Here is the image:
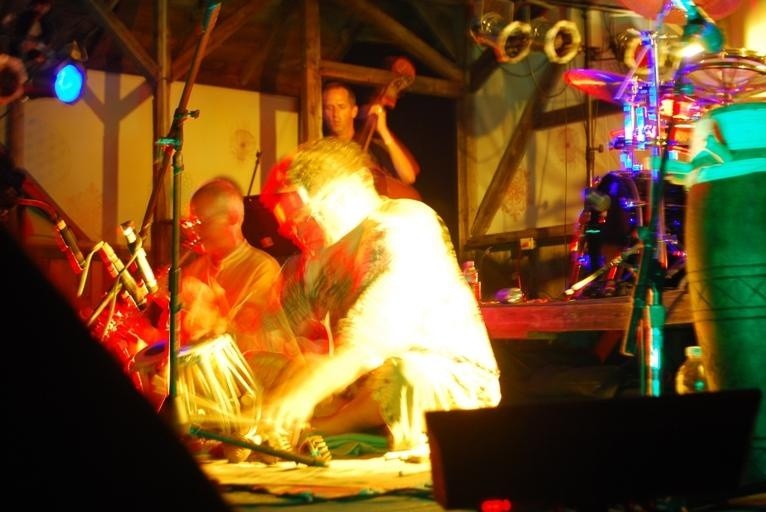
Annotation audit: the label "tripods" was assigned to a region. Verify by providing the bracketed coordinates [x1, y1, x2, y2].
[141, 0, 326, 470]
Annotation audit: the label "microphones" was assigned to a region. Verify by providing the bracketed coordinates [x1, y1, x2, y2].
[98, 241, 147, 312]
[52, 217, 86, 270]
[121, 226, 159, 295]
[674, 0, 726, 54]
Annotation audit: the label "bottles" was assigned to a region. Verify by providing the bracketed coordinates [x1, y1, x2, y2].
[676, 345, 714, 394]
[637, 288, 667, 397]
[464, 259, 478, 303]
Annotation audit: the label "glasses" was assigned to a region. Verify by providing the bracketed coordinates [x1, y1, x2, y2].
[183, 213, 227, 229]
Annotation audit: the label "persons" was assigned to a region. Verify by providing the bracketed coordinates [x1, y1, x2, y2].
[256, 140, 501, 457]
[322, 83, 415, 187]
[179, 179, 302, 361]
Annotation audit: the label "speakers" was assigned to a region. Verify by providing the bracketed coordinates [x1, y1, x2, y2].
[424, 386, 762, 512]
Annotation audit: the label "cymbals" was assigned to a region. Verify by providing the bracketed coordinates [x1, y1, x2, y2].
[565, 68, 646, 105]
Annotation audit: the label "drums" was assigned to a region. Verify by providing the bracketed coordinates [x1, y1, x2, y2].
[677, 47, 762, 101]
[134, 332, 260, 460]
[576, 173, 687, 297]
[688, 104, 766, 481]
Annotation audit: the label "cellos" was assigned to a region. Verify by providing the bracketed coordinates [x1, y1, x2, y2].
[359, 58, 422, 204]
